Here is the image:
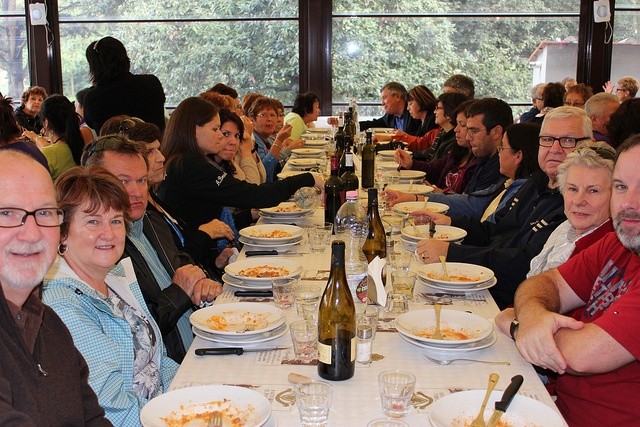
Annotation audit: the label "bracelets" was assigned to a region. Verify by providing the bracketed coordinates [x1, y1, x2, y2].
[414, 194, 418, 201]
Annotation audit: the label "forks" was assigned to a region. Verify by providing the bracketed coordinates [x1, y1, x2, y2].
[420, 293, 486, 301]
[397, 153, 402, 172]
[208, 414, 222, 427]
[423, 354, 510, 366]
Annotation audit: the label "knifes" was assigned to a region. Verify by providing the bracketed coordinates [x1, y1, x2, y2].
[429, 218, 437, 239]
[425, 292, 465, 297]
[486, 375, 524, 427]
[196, 346, 290, 355]
[245, 250, 295, 256]
[234, 290, 292, 297]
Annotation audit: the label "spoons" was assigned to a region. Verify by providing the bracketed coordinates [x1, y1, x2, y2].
[439, 256, 450, 281]
[432, 304, 444, 340]
[470, 373, 499, 427]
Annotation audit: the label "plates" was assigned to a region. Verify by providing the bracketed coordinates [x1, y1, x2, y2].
[416, 274, 497, 295]
[224, 257, 303, 285]
[427, 390, 566, 427]
[400, 225, 467, 241]
[287, 158, 322, 166]
[292, 153, 322, 158]
[393, 202, 450, 214]
[238, 236, 303, 251]
[377, 154, 413, 160]
[417, 262, 495, 288]
[139, 384, 272, 427]
[303, 144, 326, 147]
[259, 202, 311, 218]
[394, 309, 494, 347]
[307, 128, 331, 132]
[276, 171, 320, 180]
[398, 328, 497, 353]
[372, 132, 397, 141]
[191, 322, 290, 343]
[291, 149, 324, 154]
[377, 150, 413, 156]
[288, 165, 321, 169]
[387, 184, 434, 195]
[189, 302, 286, 336]
[384, 169, 426, 178]
[376, 141, 408, 145]
[258, 213, 309, 224]
[399, 235, 465, 244]
[239, 224, 305, 244]
[303, 140, 329, 144]
[222, 272, 301, 292]
[366, 127, 395, 133]
[378, 161, 401, 170]
[393, 211, 448, 218]
[392, 176, 426, 184]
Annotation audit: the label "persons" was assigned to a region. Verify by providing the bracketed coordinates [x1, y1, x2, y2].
[153, 97, 324, 250]
[21, 86, 99, 149]
[495, 135, 640, 427]
[99, 115, 238, 287]
[0, 94, 51, 178]
[563, 83, 593, 108]
[0, 148, 115, 427]
[282, 93, 321, 146]
[410, 92, 469, 164]
[80, 134, 222, 362]
[584, 92, 621, 149]
[560, 77, 577, 93]
[41, 166, 182, 427]
[200, 92, 267, 213]
[38, 94, 84, 183]
[528, 82, 565, 122]
[209, 83, 245, 117]
[525, 140, 619, 281]
[327, 83, 410, 131]
[83, 37, 165, 138]
[405, 85, 440, 137]
[416, 105, 593, 311]
[268, 100, 305, 163]
[380, 123, 541, 242]
[247, 97, 293, 184]
[13, 85, 49, 135]
[520, 83, 548, 123]
[460, 98, 515, 195]
[602, 77, 638, 104]
[391, 74, 475, 152]
[607, 98, 639, 149]
[206, 108, 261, 252]
[394, 99, 481, 195]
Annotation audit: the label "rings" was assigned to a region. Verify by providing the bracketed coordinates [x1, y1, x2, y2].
[421, 253, 426, 261]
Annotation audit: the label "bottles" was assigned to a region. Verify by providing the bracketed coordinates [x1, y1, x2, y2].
[324, 156, 344, 231]
[349, 107, 354, 114]
[340, 152, 359, 202]
[358, 187, 386, 287]
[293, 185, 324, 209]
[335, 112, 348, 151]
[385, 239, 395, 265]
[349, 112, 356, 137]
[334, 190, 370, 315]
[343, 114, 354, 145]
[339, 136, 355, 178]
[355, 326, 373, 362]
[362, 131, 374, 188]
[317, 240, 356, 381]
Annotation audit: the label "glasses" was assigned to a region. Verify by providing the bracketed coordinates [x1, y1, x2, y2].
[563, 100, 585, 107]
[538, 135, 590, 148]
[591, 145, 617, 161]
[496, 145, 512, 151]
[256, 112, 279, 118]
[616, 88, 627, 92]
[119, 116, 145, 136]
[435, 106, 444, 110]
[84, 136, 115, 165]
[0, 207, 66, 228]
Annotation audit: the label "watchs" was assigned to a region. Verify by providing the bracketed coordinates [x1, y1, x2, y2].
[510, 317, 520, 342]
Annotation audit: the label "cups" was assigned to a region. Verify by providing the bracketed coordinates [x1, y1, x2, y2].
[384, 291, 409, 315]
[355, 305, 379, 341]
[271, 277, 296, 310]
[366, 417, 410, 427]
[390, 270, 417, 300]
[289, 320, 320, 362]
[378, 370, 417, 418]
[294, 284, 321, 321]
[389, 251, 413, 274]
[295, 380, 333, 427]
[314, 222, 333, 245]
[307, 227, 326, 254]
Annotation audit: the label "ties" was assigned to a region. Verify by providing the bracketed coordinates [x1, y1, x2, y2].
[480, 178, 513, 222]
